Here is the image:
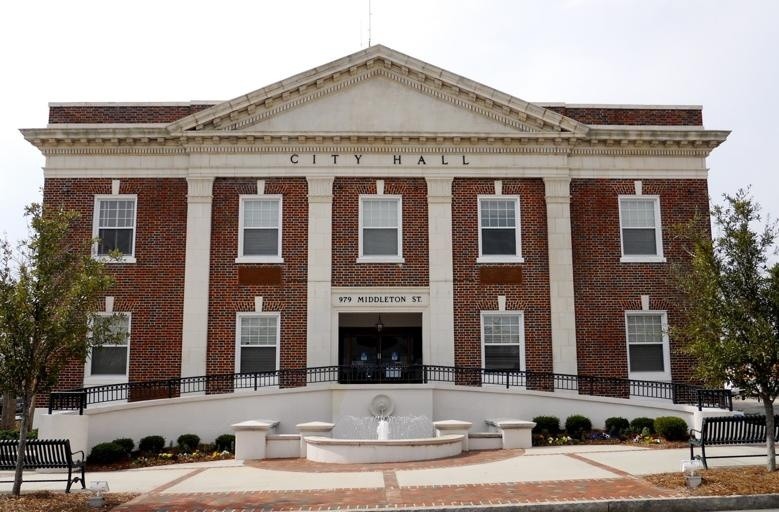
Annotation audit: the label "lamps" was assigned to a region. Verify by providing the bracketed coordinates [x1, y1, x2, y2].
[375, 313, 384, 332]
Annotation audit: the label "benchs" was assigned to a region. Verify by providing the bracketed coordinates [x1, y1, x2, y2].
[0, 439, 85, 493]
[689, 415, 778, 470]
